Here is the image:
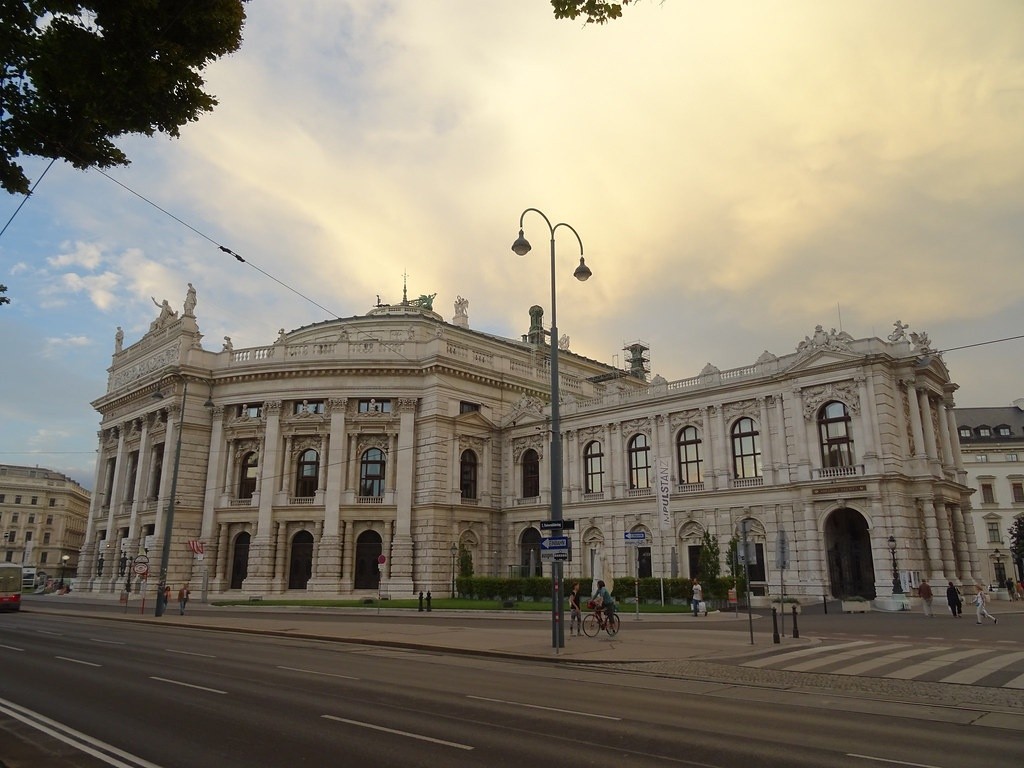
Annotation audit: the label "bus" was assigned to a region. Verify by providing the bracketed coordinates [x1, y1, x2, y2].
[0, 562, 23, 610]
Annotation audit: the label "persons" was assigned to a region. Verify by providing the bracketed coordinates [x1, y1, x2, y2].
[178, 585, 191, 616]
[590, 580, 616, 629]
[692, 578, 707, 617]
[970, 584, 998, 625]
[918, 579, 936, 618]
[151, 295, 175, 330]
[1005, 577, 1024, 602]
[51, 579, 70, 593]
[568, 582, 584, 637]
[946, 582, 962, 618]
[183, 282, 197, 316]
[114, 326, 124, 355]
[277, 328, 287, 345]
[454, 297, 469, 316]
[223, 337, 233, 352]
[161, 585, 170, 615]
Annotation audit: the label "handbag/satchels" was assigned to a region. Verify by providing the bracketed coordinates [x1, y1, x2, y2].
[691, 604, 693, 610]
[699, 602, 706, 612]
[955, 588, 963, 601]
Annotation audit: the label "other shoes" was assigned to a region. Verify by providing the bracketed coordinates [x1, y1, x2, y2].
[994, 619, 998, 624]
[976, 622, 982, 625]
[692, 614, 697, 616]
[705, 612, 707, 616]
[577, 633, 584, 636]
[570, 634, 577, 637]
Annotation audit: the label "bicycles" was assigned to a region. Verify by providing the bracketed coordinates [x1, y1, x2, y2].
[582, 602, 621, 638]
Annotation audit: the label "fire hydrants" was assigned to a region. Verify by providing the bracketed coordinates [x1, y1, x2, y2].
[426, 591, 433, 612]
[418, 591, 424, 612]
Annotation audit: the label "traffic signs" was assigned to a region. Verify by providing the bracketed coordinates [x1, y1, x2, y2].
[539, 537, 567, 549]
[624, 532, 646, 540]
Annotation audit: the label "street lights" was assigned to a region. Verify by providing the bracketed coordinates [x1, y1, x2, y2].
[152, 371, 216, 617]
[1001, 535, 1018, 583]
[887, 535, 904, 595]
[450, 542, 458, 599]
[56, 555, 70, 590]
[994, 548, 1007, 589]
[513, 209, 593, 645]
[124, 556, 133, 592]
